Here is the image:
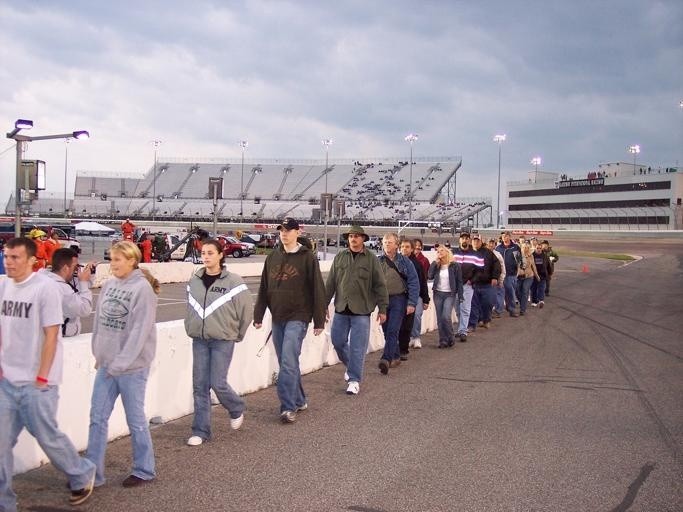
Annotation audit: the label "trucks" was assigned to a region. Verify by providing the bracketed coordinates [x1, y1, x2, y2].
[28, 228, 80, 255]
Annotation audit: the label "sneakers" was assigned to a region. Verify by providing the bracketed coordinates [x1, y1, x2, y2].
[468, 294, 551, 334]
[64, 463, 99, 506]
[437, 340, 455, 349]
[279, 402, 308, 425]
[185, 434, 207, 447]
[454, 333, 468, 343]
[343, 368, 361, 395]
[378, 352, 409, 376]
[229, 413, 244, 432]
[408, 336, 422, 349]
[121, 474, 143, 489]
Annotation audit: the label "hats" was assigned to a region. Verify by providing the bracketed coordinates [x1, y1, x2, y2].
[455, 230, 550, 248]
[276, 216, 300, 233]
[341, 223, 371, 244]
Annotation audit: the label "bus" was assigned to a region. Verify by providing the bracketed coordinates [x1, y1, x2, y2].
[0, 222, 75, 240]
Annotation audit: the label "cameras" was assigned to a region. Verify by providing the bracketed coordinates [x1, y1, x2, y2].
[77, 264, 96, 274]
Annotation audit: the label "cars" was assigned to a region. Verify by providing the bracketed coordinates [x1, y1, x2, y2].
[103, 227, 282, 263]
[315, 233, 383, 254]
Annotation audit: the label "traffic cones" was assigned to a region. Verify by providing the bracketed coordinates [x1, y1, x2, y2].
[581, 263, 588, 272]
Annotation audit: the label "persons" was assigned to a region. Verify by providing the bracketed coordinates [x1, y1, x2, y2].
[28, 228, 95, 338]
[0, 237, 96, 512]
[66, 241, 157, 488]
[253, 218, 327, 423]
[183, 240, 254, 447]
[121, 217, 169, 264]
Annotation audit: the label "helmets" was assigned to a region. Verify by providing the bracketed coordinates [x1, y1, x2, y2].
[34, 230, 46, 238]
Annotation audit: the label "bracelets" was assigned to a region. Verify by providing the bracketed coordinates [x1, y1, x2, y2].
[35, 375, 49, 384]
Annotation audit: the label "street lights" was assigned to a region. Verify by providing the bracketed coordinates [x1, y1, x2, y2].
[236, 139, 248, 221]
[629, 144, 640, 176]
[321, 137, 333, 222]
[150, 137, 162, 222]
[490, 132, 506, 229]
[402, 133, 417, 221]
[62, 138, 73, 214]
[531, 157, 543, 184]
